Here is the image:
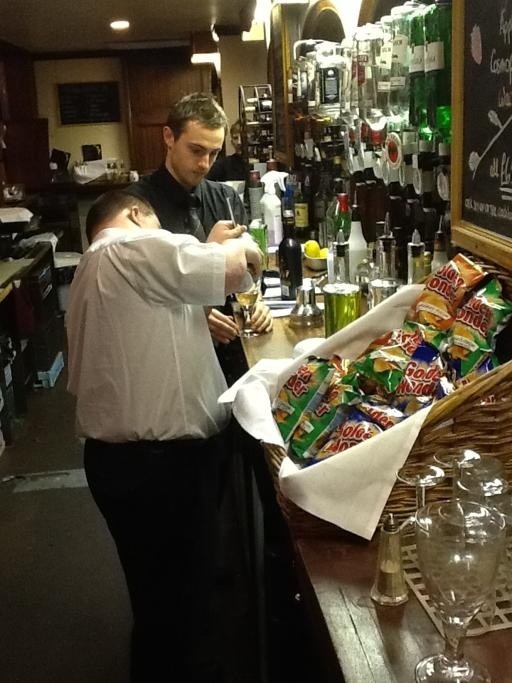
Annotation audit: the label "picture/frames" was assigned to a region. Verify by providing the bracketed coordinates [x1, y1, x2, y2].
[270, 3, 294, 166]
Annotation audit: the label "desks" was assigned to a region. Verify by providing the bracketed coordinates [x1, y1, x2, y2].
[232, 251, 512, 683]
[0, 257, 34, 446]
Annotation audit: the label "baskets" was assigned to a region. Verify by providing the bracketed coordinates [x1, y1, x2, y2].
[263, 255, 511, 537]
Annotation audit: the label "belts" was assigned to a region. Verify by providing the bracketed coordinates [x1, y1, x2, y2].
[87, 432, 227, 449]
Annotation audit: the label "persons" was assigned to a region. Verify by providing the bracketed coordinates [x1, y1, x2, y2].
[66, 188, 264, 682]
[137, 91, 276, 387]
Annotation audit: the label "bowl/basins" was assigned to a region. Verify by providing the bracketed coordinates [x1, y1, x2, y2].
[303, 249, 328, 271]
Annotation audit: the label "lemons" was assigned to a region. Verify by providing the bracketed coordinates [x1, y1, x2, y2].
[304, 240, 328, 258]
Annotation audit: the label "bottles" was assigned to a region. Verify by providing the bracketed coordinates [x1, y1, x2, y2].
[325, 192, 447, 287]
[293, 2, 459, 188]
[368, 509, 410, 610]
[277, 209, 303, 302]
[282, 180, 312, 239]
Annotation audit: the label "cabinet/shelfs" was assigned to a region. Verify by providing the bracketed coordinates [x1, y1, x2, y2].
[238, 84, 272, 159]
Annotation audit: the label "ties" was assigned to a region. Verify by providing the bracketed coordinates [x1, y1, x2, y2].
[184, 198, 206, 246]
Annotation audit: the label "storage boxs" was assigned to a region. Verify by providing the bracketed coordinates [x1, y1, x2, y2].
[10, 242, 83, 391]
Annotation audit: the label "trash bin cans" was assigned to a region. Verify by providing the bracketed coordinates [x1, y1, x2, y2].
[53, 252, 84, 312]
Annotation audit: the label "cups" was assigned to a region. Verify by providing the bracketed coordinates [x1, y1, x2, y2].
[368, 279, 398, 308]
[322, 282, 362, 339]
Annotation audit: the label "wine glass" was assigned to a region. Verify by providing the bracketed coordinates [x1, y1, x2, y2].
[234, 281, 263, 339]
[410, 498, 509, 682]
